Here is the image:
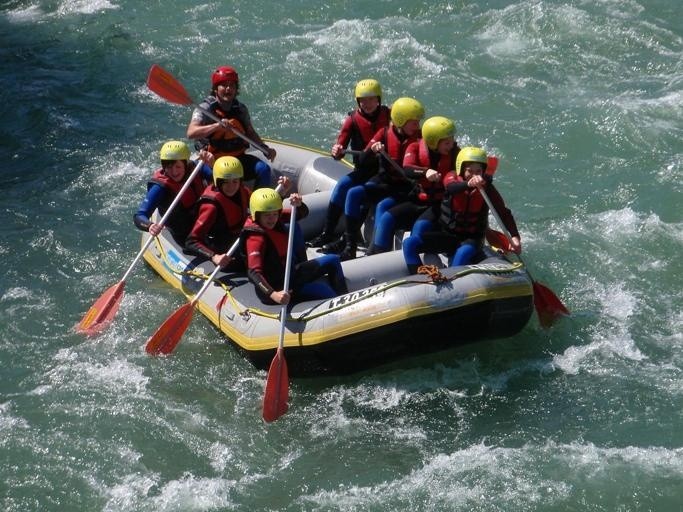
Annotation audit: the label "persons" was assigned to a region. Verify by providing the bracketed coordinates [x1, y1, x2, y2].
[240, 188, 347, 305]
[365, 116, 461, 257]
[337, 97, 425, 260]
[184, 155, 292, 270]
[401, 146, 522, 275]
[132, 141, 215, 249]
[305, 79, 391, 254]
[186, 66, 276, 190]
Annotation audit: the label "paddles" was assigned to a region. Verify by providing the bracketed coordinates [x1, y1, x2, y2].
[78, 160, 203, 330]
[437, 174, 511, 251]
[146, 63, 273, 158]
[476, 185, 571, 331]
[146, 181, 286, 358]
[262, 202, 297, 422]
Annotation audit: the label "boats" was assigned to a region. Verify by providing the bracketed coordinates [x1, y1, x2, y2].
[140, 137, 534, 377]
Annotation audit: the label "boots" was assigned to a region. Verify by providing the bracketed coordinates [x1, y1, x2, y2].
[322, 208, 369, 252]
[305, 200, 342, 248]
[340, 215, 361, 260]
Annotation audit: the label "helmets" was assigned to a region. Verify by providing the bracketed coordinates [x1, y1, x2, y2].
[212, 155, 244, 187]
[390, 98, 425, 127]
[160, 141, 189, 161]
[355, 79, 382, 97]
[456, 147, 488, 176]
[212, 66, 238, 84]
[422, 116, 456, 150]
[249, 188, 283, 221]
